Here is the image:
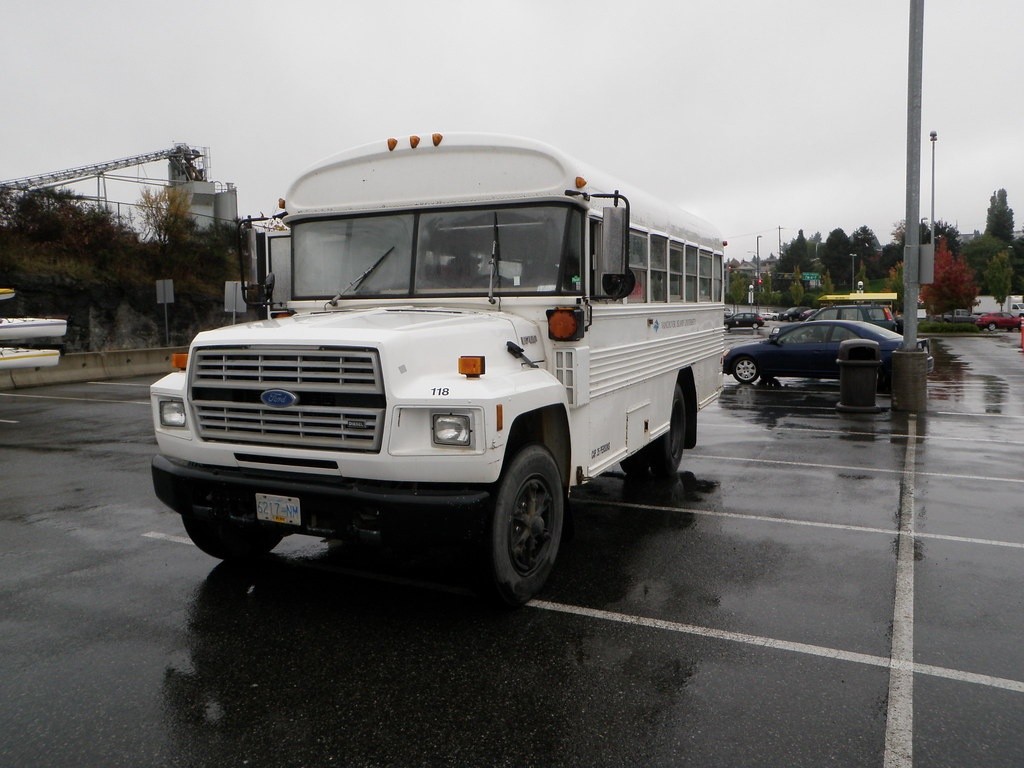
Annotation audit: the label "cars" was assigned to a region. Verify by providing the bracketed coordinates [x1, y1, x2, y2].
[724, 312, 765, 331]
[721, 320, 935, 388]
[799, 308, 819, 322]
[723, 306, 733, 319]
[762, 311, 779, 321]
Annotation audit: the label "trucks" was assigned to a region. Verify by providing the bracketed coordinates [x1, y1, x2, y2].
[971, 295, 1024, 318]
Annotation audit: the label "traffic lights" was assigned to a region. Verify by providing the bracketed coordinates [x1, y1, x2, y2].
[758, 278, 763, 284]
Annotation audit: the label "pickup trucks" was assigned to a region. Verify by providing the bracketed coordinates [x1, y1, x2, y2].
[935, 309, 981, 324]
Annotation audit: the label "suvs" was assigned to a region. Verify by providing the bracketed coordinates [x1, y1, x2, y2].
[976, 311, 1022, 331]
[771, 304, 897, 341]
[778, 306, 811, 321]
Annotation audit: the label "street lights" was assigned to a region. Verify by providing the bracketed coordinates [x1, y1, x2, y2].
[849, 253, 857, 293]
[928, 130, 938, 246]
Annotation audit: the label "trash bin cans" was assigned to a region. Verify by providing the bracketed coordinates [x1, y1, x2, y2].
[834, 338, 884, 413]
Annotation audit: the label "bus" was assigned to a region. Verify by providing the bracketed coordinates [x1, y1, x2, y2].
[146, 134, 731, 599]
